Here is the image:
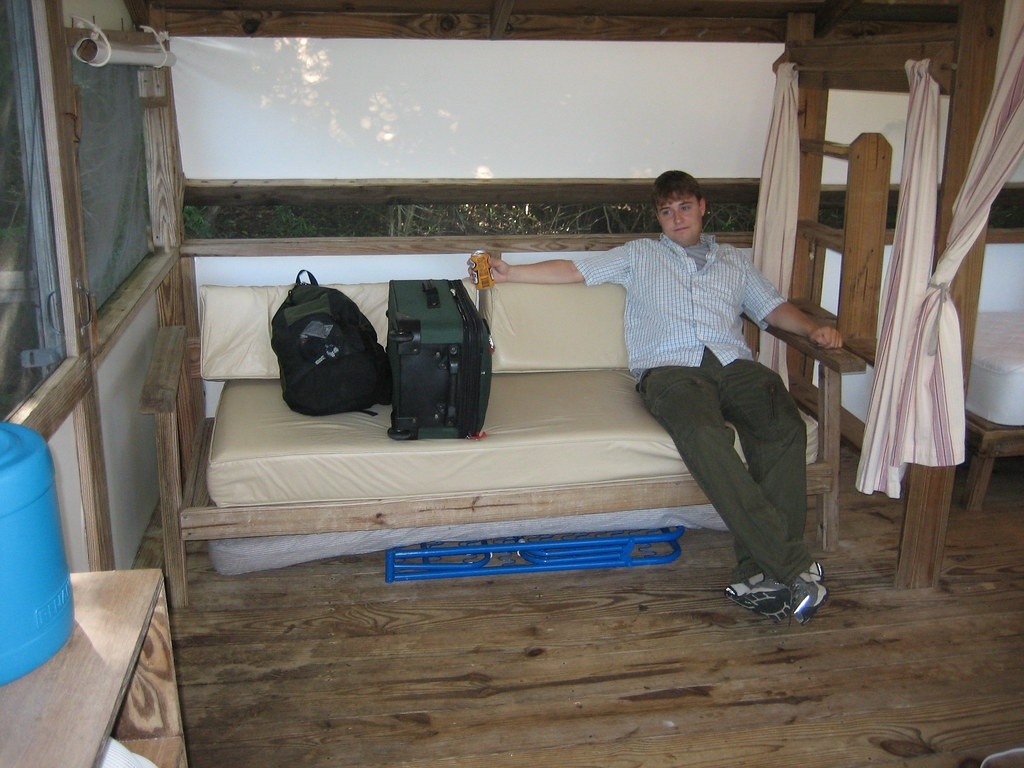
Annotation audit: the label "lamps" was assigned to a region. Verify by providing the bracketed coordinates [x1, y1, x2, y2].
[71, 14, 176, 70]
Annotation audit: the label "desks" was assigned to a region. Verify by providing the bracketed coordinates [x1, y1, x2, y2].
[0, 569, 188, 768]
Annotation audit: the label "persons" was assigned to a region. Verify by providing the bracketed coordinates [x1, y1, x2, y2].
[468, 171, 844, 624]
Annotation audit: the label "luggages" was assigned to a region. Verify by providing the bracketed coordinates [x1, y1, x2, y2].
[385, 279, 496, 440]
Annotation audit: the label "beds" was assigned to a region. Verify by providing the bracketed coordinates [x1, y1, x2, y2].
[809, 242, 1024, 509]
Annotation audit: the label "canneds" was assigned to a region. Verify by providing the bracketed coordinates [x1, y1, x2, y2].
[471, 250, 495, 289]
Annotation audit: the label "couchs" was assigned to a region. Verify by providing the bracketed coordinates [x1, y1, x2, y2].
[141, 282, 870, 608]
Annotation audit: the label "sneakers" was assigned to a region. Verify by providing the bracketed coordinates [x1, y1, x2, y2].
[724, 559, 795, 621]
[775, 561, 830, 626]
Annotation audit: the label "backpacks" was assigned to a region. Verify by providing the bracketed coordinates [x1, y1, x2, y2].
[271, 270, 386, 416]
